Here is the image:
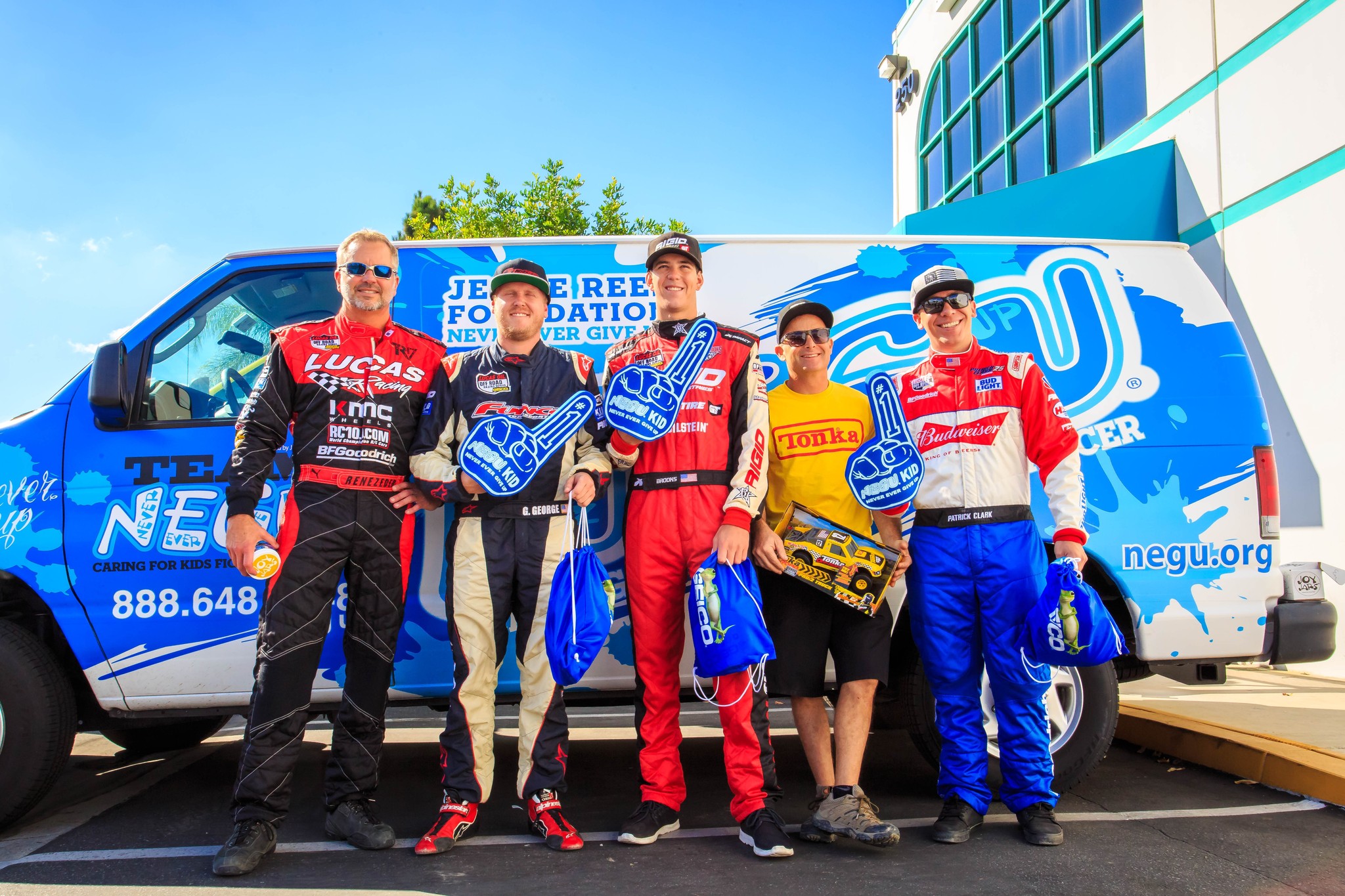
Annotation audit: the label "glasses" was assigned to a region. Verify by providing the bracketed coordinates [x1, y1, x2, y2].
[780, 328, 831, 347]
[916, 293, 972, 314]
[335, 263, 398, 280]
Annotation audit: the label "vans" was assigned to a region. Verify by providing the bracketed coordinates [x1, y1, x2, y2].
[0, 236, 1336, 835]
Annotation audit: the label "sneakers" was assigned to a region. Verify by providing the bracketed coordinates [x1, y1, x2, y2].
[737, 807, 794, 856]
[1017, 802, 1063, 844]
[812, 784, 900, 846]
[325, 798, 394, 849]
[527, 788, 584, 851]
[932, 794, 984, 843]
[615, 799, 681, 844]
[212, 817, 278, 875]
[799, 785, 835, 844]
[413, 790, 477, 854]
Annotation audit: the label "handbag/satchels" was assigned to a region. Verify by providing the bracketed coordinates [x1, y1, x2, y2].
[688, 545, 775, 707]
[1014, 557, 1129, 667]
[546, 490, 616, 685]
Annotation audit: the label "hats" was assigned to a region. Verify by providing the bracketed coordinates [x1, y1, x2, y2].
[491, 259, 551, 303]
[645, 230, 702, 271]
[910, 265, 974, 314]
[776, 299, 833, 344]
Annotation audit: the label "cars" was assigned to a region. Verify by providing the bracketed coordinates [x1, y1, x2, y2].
[783, 526, 887, 595]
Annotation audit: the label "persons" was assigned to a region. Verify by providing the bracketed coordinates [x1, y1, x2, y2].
[604, 230, 793, 859]
[744, 299, 913, 845]
[874, 265, 1088, 846]
[407, 257, 615, 856]
[211, 230, 448, 876]
